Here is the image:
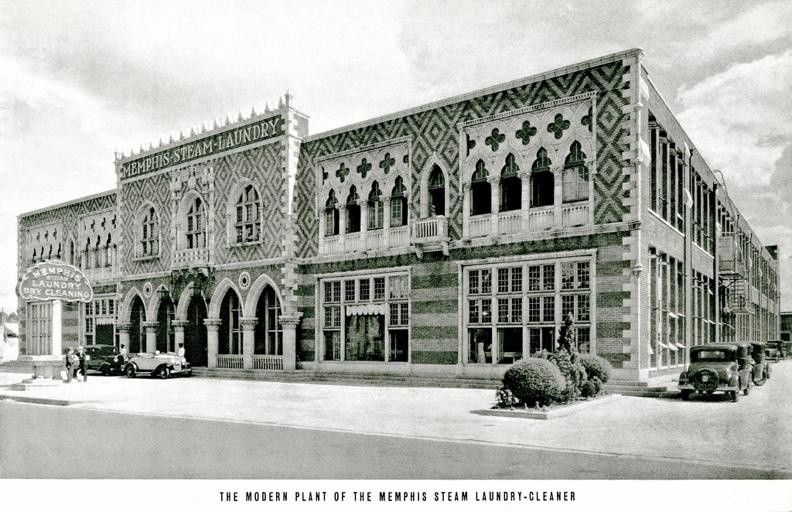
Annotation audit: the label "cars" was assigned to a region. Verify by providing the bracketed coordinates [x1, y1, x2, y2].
[122, 351, 193, 378]
[66, 344, 124, 375]
[678, 340, 791, 402]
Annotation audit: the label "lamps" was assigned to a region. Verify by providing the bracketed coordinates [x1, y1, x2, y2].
[156, 284, 180, 308]
[188, 281, 210, 304]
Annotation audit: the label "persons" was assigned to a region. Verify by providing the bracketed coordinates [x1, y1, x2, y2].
[117, 344, 129, 362]
[63, 345, 89, 383]
[176, 342, 187, 369]
